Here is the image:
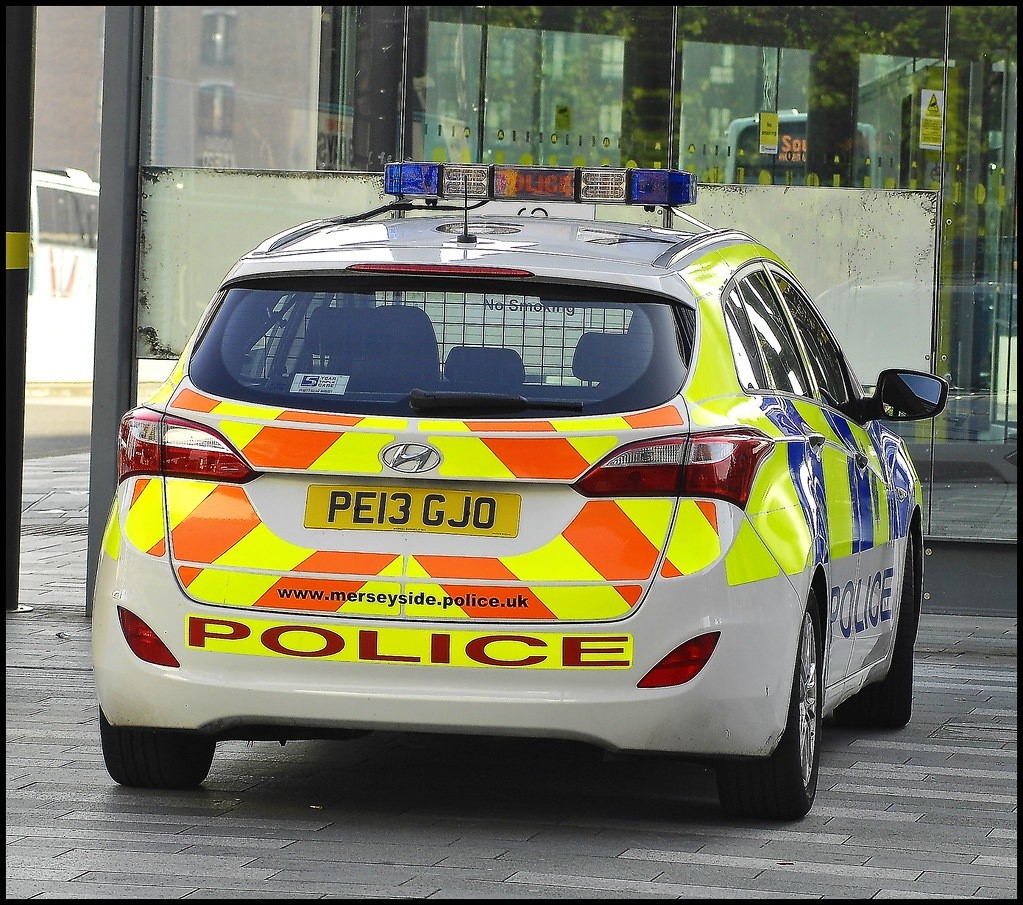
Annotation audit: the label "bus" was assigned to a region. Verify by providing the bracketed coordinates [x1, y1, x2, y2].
[722, 108, 885, 190]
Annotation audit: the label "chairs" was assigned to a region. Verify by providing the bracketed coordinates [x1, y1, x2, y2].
[443, 346, 523, 393]
[303, 306, 437, 393]
[572, 332, 632, 388]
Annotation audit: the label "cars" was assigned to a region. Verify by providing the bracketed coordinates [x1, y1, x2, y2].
[90, 160, 948, 822]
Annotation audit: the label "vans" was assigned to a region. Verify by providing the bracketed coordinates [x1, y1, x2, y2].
[30, 167, 98, 386]
